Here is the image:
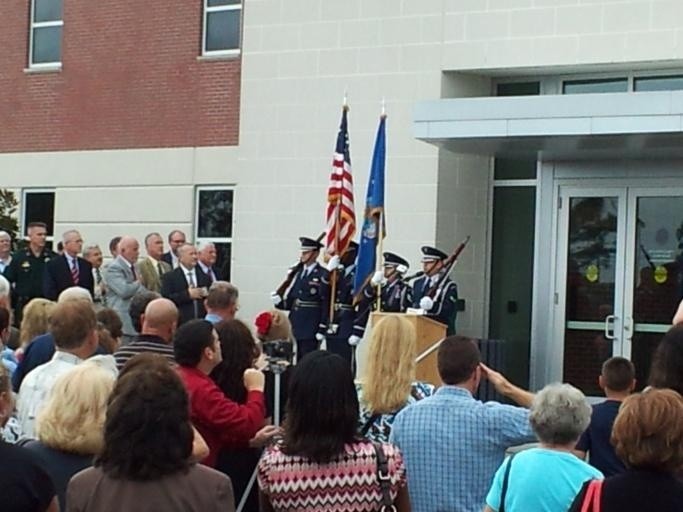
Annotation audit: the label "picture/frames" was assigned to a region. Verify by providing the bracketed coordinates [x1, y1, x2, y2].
[372, 311, 449, 391]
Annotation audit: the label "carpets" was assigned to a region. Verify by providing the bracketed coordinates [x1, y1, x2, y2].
[208, 268, 213, 282]
[96, 268, 105, 295]
[130, 266, 136, 280]
[422, 279, 431, 295]
[157, 263, 162, 277]
[188, 272, 195, 288]
[70, 259, 79, 285]
[303, 270, 307, 279]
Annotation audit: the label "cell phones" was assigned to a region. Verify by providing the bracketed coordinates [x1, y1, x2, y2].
[262, 339, 293, 358]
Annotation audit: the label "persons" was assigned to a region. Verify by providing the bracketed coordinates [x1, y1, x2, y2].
[567, 389, 683, 512]
[16, 364, 115, 505]
[257, 350, 408, 512]
[15, 296, 56, 362]
[389, 335, 536, 512]
[254, 310, 295, 428]
[109, 237, 122, 259]
[160, 243, 210, 325]
[210, 319, 261, 405]
[413, 245, 457, 323]
[105, 237, 147, 336]
[112, 298, 179, 373]
[361, 251, 413, 313]
[82, 244, 109, 300]
[204, 281, 240, 329]
[128, 291, 163, 334]
[0, 273, 20, 349]
[0, 230, 15, 273]
[11, 286, 106, 395]
[138, 233, 172, 291]
[2, 222, 59, 309]
[0, 307, 15, 369]
[270, 237, 330, 362]
[325, 240, 371, 377]
[96, 308, 123, 348]
[197, 243, 219, 286]
[648, 320, 683, 397]
[65, 353, 235, 512]
[16, 300, 100, 440]
[43, 230, 94, 300]
[162, 229, 185, 264]
[171, 319, 265, 468]
[0, 363, 61, 511]
[482, 382, 605, 512]
[571, 357, 636, 476]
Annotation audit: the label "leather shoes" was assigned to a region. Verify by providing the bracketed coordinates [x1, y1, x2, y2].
[370, 271, 384, 285]
[271, 292, 281, 305]
[316, 333, 323, 340]
[348, 335, 360, 345]
[419, 295, 433, 310]
[326, 254, 340, 272]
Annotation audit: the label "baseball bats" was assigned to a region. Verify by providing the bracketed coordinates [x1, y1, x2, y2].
[276, 232, 326, 296]
[427, 235, 470, 298]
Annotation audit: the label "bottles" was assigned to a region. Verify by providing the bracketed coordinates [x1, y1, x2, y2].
[298, 236, 324, 253]
[347, 241, 359, 253]
[420, 245, 448, 263]
[382, 252, 409, 269]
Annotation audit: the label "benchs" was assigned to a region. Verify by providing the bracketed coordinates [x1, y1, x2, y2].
[324, 106, 356, 272]
[353, 115, 387, 288]
